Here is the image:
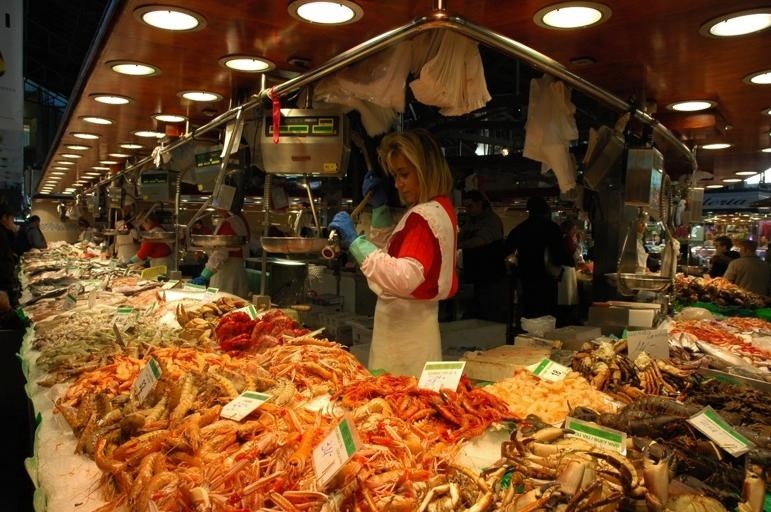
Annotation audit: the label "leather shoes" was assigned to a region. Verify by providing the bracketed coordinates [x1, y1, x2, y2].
[127, 255, 138, 264]
[362, 171, 394, 229]
[327, 211, 379, 266]
[191, 266, 213, 285]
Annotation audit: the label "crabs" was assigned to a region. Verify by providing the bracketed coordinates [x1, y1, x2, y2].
[414, 413, 770, 512]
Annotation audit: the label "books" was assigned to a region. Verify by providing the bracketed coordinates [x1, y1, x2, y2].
[440, 318, 509, 353]
[347, 315, 374, 342]
[564, 324, 603, 341]
[588, 305, 656, 328]
[350, 332, 460, 373]
[244, 255, 308, 303]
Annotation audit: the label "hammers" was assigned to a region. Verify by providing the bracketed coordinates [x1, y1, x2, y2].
[565, 396, 771, 498]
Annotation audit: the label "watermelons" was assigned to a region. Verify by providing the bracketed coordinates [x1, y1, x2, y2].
[186, 145, 248, 252]
[260, 108, 351, 254]
[102, 187, 129, 235]
[140, 170, 177, 240]
[602, 146, 675, 297]
[676, 187, 704, 275]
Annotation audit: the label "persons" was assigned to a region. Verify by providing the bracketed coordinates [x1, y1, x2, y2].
[719, 239, 770, 296]
[503, 193, 567, 334]
[192, 219, 212, 235]
[446, 188, 515, 336]
[707, 236, 741, 279]
[0, 200, 49, 322]
[72, 211, 174, 271]
[186, 207, 250, 302]
[555, 217, 595, 333]
[325, 126, 460, 391]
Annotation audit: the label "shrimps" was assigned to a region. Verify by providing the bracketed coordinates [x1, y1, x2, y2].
[51, 296, 521, 512]
[565, 396, 771, 498]
